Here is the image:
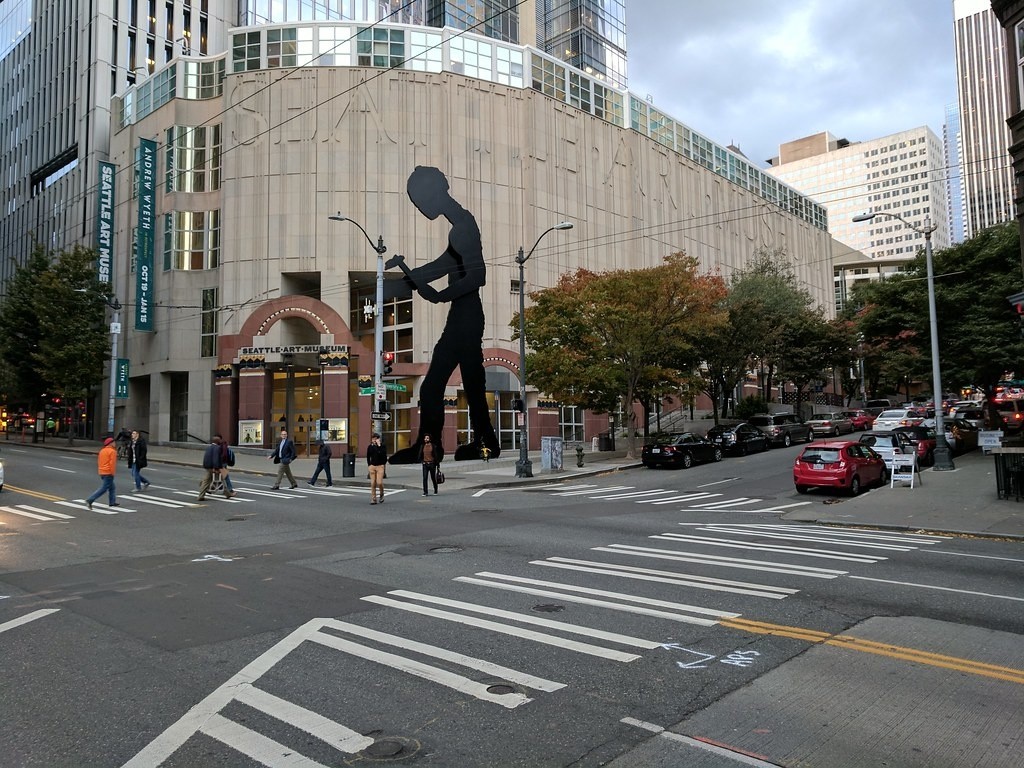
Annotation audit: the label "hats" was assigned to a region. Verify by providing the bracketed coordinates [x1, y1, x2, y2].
[104, 438, 114, 446]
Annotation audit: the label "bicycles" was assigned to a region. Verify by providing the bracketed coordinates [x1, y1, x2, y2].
[117, 443, 132, 460]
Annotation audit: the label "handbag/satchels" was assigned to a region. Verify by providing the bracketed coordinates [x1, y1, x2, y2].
[437, 470, 445, 484]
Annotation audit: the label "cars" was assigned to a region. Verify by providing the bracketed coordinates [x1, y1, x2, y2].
[640, 432, 722, 469]
[872, 410, 925, 432]
[807, 412, 855, 436]
[892, 425, 951, 465]
[793, 440, 888, 496]
[901, 386, 1024, 450]
[842, 409, 876, 430]
[859, 430, 919, 475]
[705, 422, 773, 459]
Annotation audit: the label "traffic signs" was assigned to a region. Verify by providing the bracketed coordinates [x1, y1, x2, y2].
[370, 412, 392, 422]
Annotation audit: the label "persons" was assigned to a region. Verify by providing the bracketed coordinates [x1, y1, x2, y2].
[197, 433, 237, 501]
[127, 430, 150, 493]
[267, 431, 298, 489]
[307, 440, 332, 487]
[366, 433, 387, 505]
[418, 434, 440, 497]
[115, 427, 130, 456]
[85, 438, 120, 510]
[46, 417, 56, 441]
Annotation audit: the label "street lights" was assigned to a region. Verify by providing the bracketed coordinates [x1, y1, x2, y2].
[73, 289, 120, 440]
[328, 214, 386, 478]
[516, 221, 574, 478]
[851, 212, 954, 470]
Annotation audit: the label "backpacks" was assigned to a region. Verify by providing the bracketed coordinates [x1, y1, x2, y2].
[227, 447, 235, 466]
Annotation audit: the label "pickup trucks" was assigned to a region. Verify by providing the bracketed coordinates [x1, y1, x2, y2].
[861, 399, 906, 415]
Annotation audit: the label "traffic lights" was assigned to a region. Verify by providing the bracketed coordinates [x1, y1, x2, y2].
[384, 353, 393, 373]
[511, 400, 524, 410]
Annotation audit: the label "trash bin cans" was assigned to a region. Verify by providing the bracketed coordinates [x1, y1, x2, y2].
[343, 453, 356, 476]
[599, 433, 611, 451]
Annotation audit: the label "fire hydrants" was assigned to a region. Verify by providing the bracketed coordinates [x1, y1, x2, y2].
[575, 446, 586, 468]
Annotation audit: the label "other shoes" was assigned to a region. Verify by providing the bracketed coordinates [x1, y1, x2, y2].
[132, 489, 142, 493]
[370, 500, 377, 504]
[271, 487, 279, 490]
[197, 496, 205, 501]
[434, 489, 438, 495]
[227, 492, 237, 498]
[307, 481, 314, 487]
[86, 500, 92, 510]
[288, 485, 297, 489]
[143, 482, 151, 490]
[109, 503, 120, 507]
[422, 493, 428, 497]
[379, 495, 385, 502]
[326, 483, 333, 488]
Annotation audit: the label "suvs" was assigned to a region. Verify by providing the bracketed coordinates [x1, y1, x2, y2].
[748, 412, 815, 447]
